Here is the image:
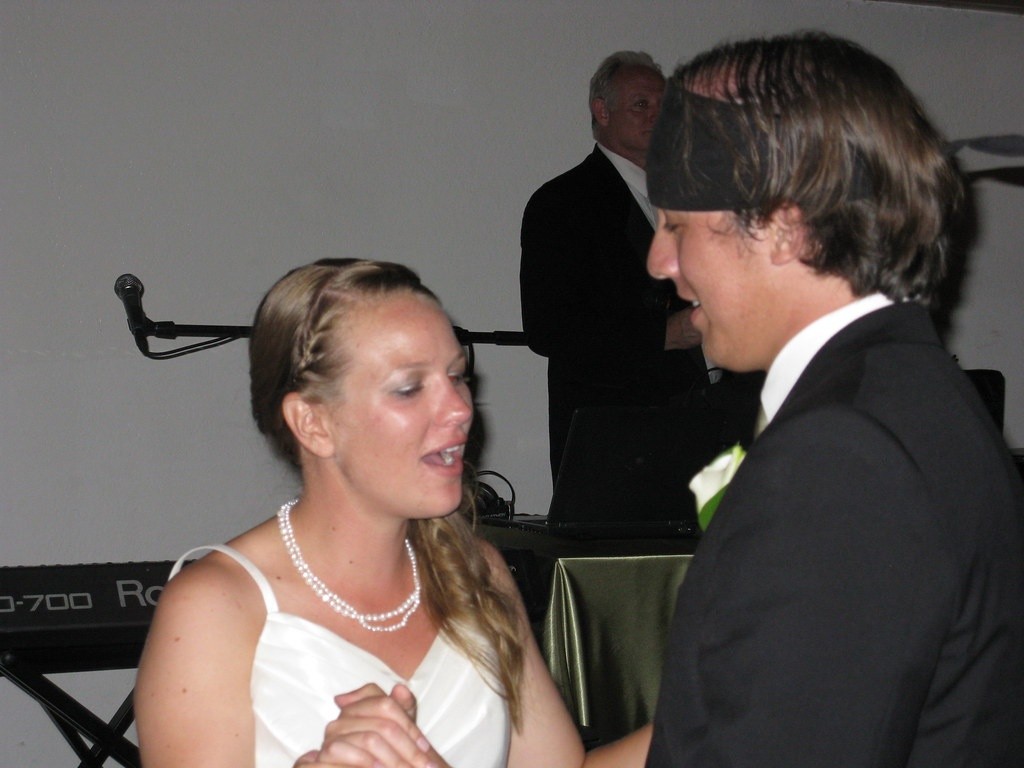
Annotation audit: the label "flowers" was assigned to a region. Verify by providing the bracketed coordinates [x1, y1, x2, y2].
[688, 441, 747, 531]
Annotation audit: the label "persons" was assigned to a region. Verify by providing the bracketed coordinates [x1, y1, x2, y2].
[520, 50, 752, 534]
[132, 30, 1024, 768]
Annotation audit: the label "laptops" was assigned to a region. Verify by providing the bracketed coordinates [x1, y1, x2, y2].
[477, 406, 729, 536]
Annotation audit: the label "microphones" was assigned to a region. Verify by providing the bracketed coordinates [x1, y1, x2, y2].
[114, 273, 148, 354]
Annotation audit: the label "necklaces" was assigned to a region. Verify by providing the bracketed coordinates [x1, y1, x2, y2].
[278, 499, 421, 633]
